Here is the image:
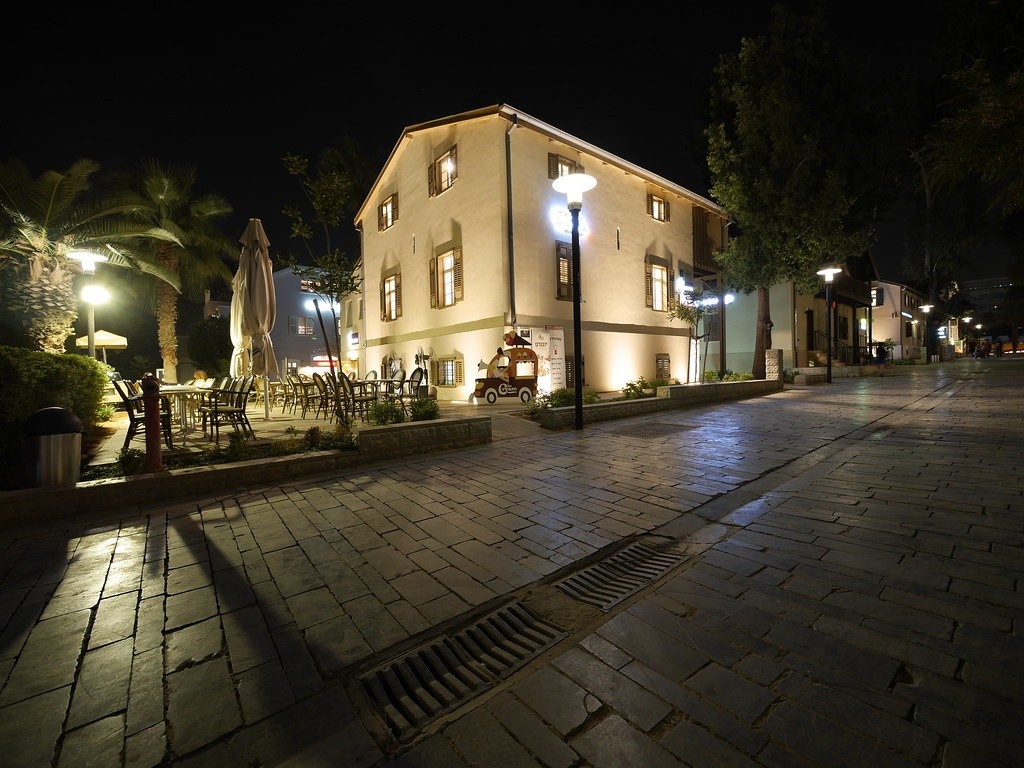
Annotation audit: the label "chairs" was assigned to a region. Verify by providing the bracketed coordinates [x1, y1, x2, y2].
[183, 367, 378, 453]
[386, 367, 424, 415]
[111, 379, 175, 450]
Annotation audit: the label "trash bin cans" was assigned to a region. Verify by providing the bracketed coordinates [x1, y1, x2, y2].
[413, 386, 429, 398]
[38, 432, 81, 487]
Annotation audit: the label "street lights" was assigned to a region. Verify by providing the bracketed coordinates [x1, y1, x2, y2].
[975, 322, 983, 346]
[918, 302, 936, 365]
[962, 314, 973, 357]
[553, 162, 599, 431]
[79, 282, 109, 357]
[816, 264, 843, 384]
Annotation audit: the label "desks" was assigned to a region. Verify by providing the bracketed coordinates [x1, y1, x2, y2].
[250, 379, 418, 420]
[157, 385, 214, 445]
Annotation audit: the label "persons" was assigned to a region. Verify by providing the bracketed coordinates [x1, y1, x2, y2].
[1012, 336, 1019, 357]
[969, 337, 1003, 358]
[876, 342, 887, 377]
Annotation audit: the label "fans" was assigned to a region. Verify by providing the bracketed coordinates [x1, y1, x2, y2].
[415, 345, 430, 385]
[388, 351, 402, 392]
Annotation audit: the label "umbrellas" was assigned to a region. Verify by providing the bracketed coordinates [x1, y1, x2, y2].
[230, 268, 252, 381]
[238, 218, 281, 418]
[76, 329, 128, 364]
